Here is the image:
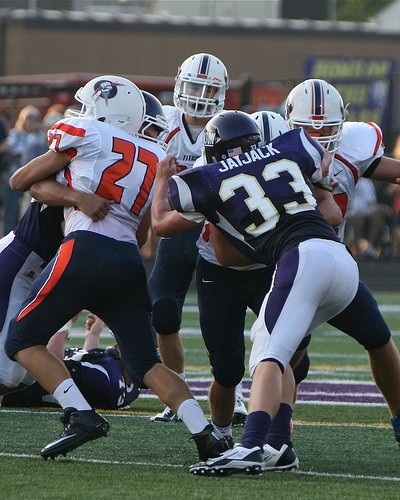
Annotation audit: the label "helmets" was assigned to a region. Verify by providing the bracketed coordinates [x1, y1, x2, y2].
[64, 76, 146, 134]
[250, 111, 288, 146]
[173, 53, 229, 118]
[204, 110, 262, 164]
[285, 80, 344, 154]
[136, 91, 169, 149]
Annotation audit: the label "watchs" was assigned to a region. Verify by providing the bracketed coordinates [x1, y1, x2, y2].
[0, 50, 400, 472]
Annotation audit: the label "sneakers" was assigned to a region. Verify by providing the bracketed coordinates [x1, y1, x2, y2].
[190, 424, 231, 462]
[263, 443, 301, 471]
[224, 435, 234, 448]
[40, 407, 111, 460]
[189, 442, 264, 476]
[149, 407, 182, 423]
[233, 396, 249, 425]
[390, 409, 400, 445]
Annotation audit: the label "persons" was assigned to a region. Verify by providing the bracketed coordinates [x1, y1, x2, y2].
[5, 74, 230, 461]
[151, 111, 359, 479]
